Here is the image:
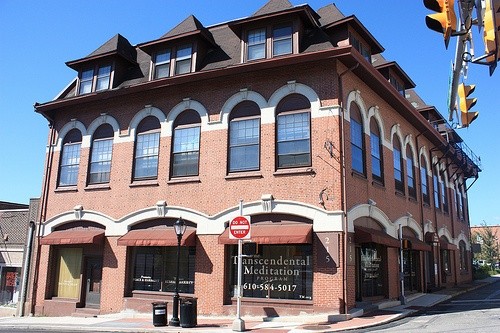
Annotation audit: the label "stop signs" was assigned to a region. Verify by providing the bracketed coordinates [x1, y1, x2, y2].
[228, 215, 250, 240]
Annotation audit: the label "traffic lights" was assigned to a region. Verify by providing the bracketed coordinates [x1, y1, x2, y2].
[422, 0, 457, 50]
[457, 82, 479, 128]
[481, 0, 500, 63]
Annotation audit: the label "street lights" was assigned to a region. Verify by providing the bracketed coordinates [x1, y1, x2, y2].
[169, 215, 187, 327]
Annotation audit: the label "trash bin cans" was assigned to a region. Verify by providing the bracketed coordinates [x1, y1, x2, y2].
[151, 301, 169, 326]
[179, 296, 198, 329]
